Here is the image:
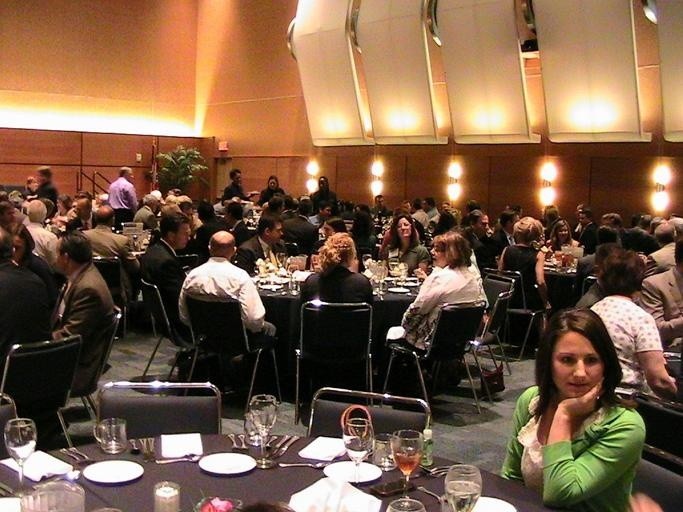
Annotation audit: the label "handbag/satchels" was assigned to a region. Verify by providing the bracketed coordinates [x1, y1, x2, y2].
[175, 348, 202, 383]
[479, 362, 506, 397]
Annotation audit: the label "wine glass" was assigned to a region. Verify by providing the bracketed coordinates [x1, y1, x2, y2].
[342, 418, 425, 507]
[369, 259, 408, 296]
[4, 419, 39, 497]
[249, 395, 278, 469]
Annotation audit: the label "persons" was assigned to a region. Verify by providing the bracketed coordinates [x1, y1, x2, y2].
[2, 165, 683, 512]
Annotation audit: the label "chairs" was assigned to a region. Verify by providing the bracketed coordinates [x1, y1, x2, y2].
[69, 303, 125, 423]
[457, 287, 517, 380]
[1, 334, 83, 446]
[0, 390, 21, 458]
[186, 291, 283, 411]
[381, 299, 489, 410]
[140, 280, 198, 375]
[581, 275, 599, 296]
[540, 262, 581, 318]
[304, 386, 430, 445]
[484, 267, 543, 362]
[295, 299, 373, 422]
[634, 436, 682, 509]
[95, 376, 225, 437]
[467, 273, 516, 362]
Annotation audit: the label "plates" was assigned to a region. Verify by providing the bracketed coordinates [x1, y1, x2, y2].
[323, 460, 382, 483]
[83, 459, 145, 484]
[261, 284, 282, 290]
[198, 453, 256, 475]
[388, 276, 420, 293]
[471, 497, 517, 512]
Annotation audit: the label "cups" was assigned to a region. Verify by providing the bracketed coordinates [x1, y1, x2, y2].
[385, 464, 483, 512]
[93, 418, 127, 455]
[20, 480, 85, 512]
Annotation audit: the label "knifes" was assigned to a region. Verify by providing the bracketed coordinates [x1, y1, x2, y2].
[265, 434, 300, 459]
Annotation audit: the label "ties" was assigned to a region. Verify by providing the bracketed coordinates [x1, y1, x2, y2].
[267, 248, 278, 268]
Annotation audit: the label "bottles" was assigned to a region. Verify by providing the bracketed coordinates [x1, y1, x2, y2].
[422, 429, 433, 466]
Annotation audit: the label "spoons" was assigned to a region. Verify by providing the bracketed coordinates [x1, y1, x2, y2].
[279, 461, 332, 469]
[129, 438, 201, 465]
[59, 447, 95, 465]
[227, 434, 248, 452]
